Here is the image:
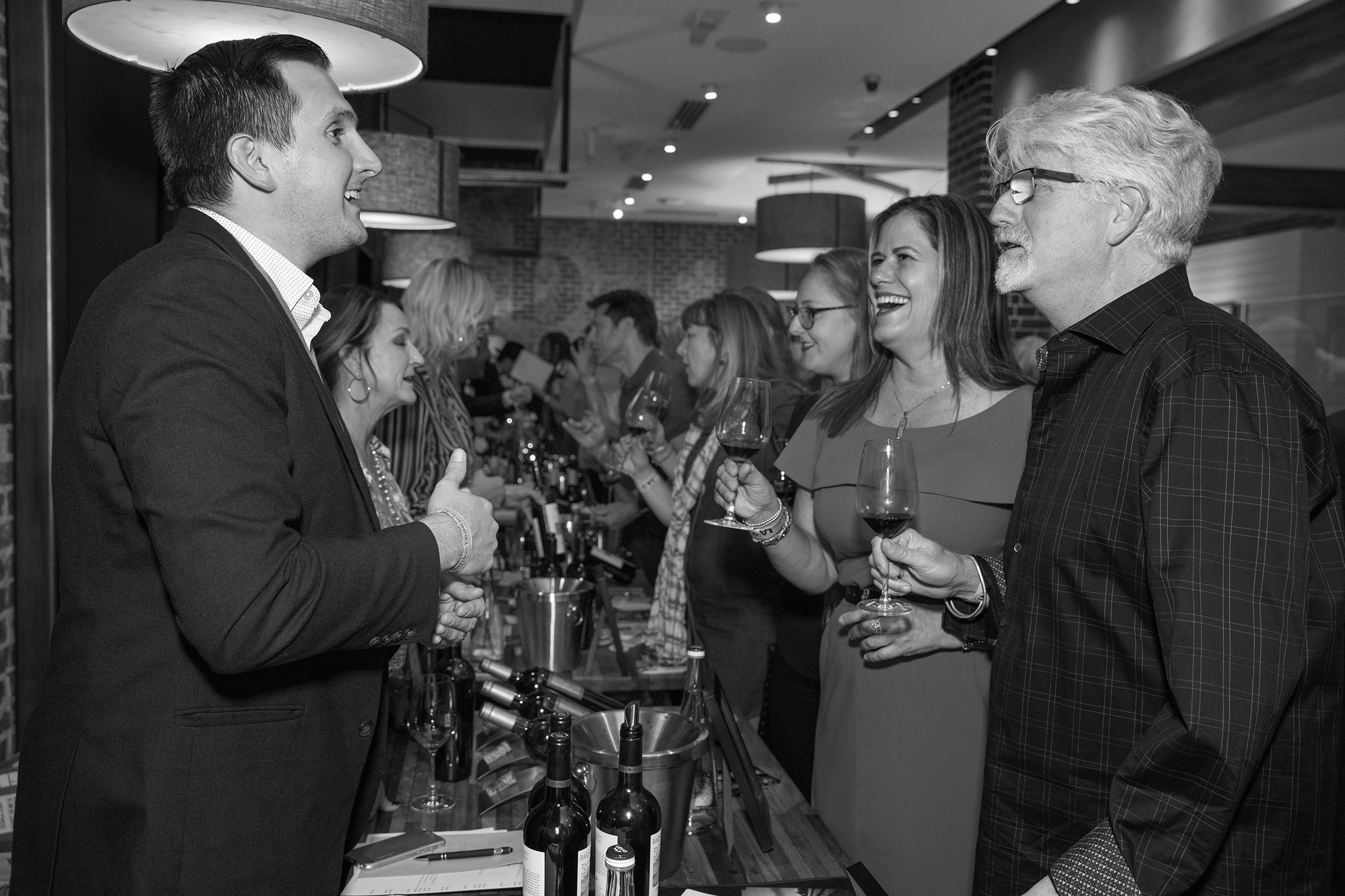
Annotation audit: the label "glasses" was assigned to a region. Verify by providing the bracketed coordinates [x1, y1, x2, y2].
[786, 306, 858, 331]
[992, 166, 1119, 205]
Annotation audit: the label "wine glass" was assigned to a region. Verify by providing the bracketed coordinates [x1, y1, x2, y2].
[604, 371, 675, 474]
[856, 438, 920, 616]
[704, 377, 772, 532]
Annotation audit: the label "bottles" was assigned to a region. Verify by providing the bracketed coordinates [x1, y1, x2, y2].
[388, 420, 718, 896]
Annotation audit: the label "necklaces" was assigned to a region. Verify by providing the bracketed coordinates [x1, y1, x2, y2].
[887, 367, 966, 440]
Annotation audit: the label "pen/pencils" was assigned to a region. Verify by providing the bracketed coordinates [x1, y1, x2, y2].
[414, 847, 513, 863]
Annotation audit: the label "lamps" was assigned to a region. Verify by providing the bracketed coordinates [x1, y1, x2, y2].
[754, 192, 868, 262]
[59, 0, 428, 93]
[342, 92, 461, 230]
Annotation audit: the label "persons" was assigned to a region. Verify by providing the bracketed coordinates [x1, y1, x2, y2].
[465, 332, 583, 463]
[563, 288, 700, 572]
[309, 282, 428, 678]
[868, 86, 1343, 896]
[603, 247, 872, 806]
[714, 193, 1037, 895]
[371, 257, 511, 524]
[15, 35, 501, 896]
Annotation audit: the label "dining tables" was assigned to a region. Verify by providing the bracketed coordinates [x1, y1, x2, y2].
[341, 540, 891, 896]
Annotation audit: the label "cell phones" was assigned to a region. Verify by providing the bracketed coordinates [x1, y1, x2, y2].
[344, 828, 445, 872]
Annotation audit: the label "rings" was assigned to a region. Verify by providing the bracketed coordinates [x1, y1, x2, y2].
[869, 618, 883, 634]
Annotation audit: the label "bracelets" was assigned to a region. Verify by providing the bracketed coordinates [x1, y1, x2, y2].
[753, 511, 792, 546]
[648, 442, 670, 467]
[428, 508, 471, 573]
[944, 555, 986, 619]
[749, 508, 786, 540]
[637, 472, 660, 492]
[742, 498, 782, 528]
